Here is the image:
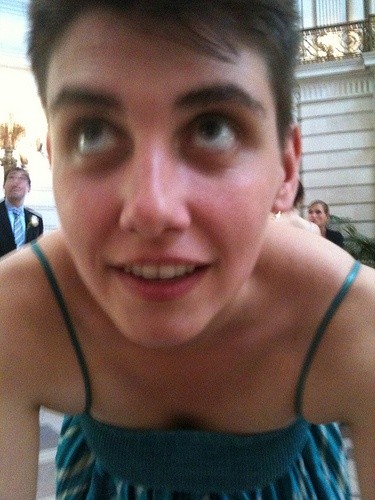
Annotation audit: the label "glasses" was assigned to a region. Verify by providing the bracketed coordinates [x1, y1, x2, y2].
[308, 209, 322, 214]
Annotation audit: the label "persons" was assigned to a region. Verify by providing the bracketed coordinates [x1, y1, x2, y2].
[1, 167, 46, 258]
[1, 0, 373, 500]
[307, 199, 344, 251]
[274, 179, 321, 237]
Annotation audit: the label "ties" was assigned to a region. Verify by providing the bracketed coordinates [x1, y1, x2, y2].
[12, 209, 23, 250]
